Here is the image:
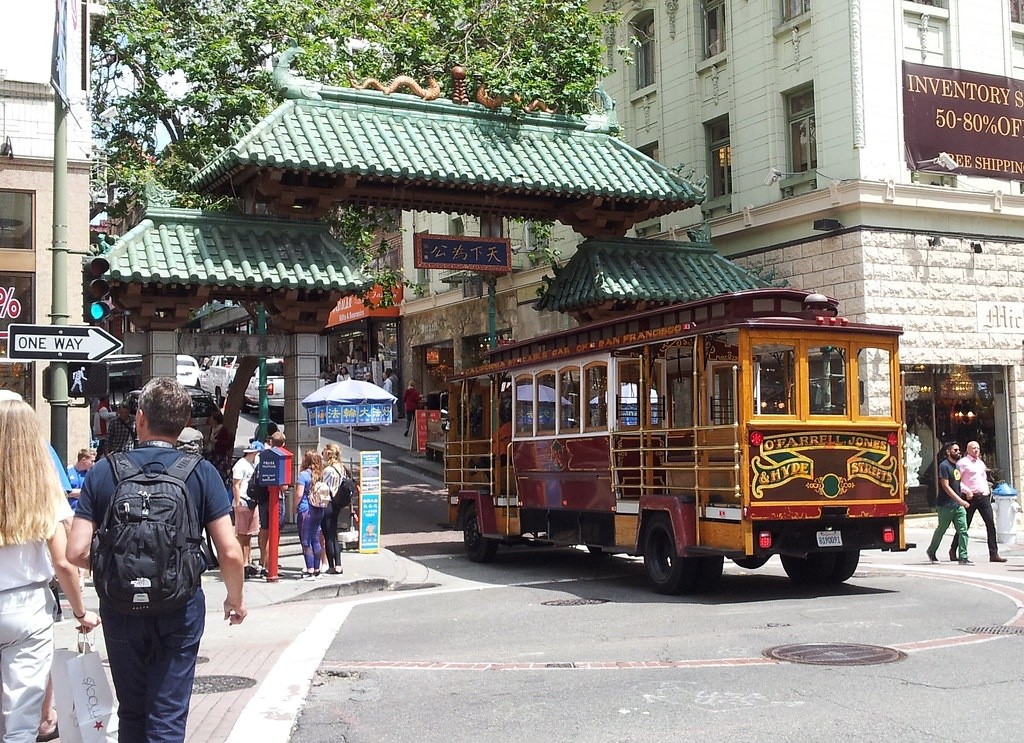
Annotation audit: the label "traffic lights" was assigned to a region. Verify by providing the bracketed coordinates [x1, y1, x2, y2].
[82, 255, 114, 324]
[68, 363, 114, 400]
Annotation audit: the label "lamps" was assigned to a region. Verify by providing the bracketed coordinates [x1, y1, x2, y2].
[814, 219, 841, 231]
[927, 236, 942, 249]
[971, 240, 983, 254]
[764, 168, 804, 186]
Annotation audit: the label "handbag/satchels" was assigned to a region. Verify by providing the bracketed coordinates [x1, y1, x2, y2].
[51, 624, 120, 743]
[242, 497, 258, 509]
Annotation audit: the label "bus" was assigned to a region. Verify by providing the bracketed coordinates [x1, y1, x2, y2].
[443, 288, 917, 596]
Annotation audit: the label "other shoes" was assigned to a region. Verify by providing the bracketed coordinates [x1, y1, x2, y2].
[315, 571, 323, 579]
[262, 569, 284, 580]
[326, 567, 344, 574]
[244, 566, 260, 578]
[296, 571, 316, 580]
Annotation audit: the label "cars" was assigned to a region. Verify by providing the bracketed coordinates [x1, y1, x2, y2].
[177, 355, 326, 429]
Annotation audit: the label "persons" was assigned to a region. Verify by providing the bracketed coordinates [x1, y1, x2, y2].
[925, 439, 1007, 565]
[230, 431, 347, 578]
[66, 376, 248, 743]
[320, 365, 420, 439]
[0, 388, 101, 743]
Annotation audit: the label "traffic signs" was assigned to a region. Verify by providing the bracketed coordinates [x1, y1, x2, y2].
[6, 323, 124, 364]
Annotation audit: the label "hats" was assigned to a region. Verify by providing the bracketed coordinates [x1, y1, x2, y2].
[244, 441, 264, 453]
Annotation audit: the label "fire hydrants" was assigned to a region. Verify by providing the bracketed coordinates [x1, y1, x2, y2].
[990, 482, 1022, 544]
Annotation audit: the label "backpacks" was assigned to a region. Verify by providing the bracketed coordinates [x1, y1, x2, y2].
[88, 452, 206, 614]
[328, 464, 356, 509]
[305, 469, 330, 508]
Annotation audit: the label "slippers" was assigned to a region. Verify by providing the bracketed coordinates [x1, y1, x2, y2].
[37, 723, 59, 742]
